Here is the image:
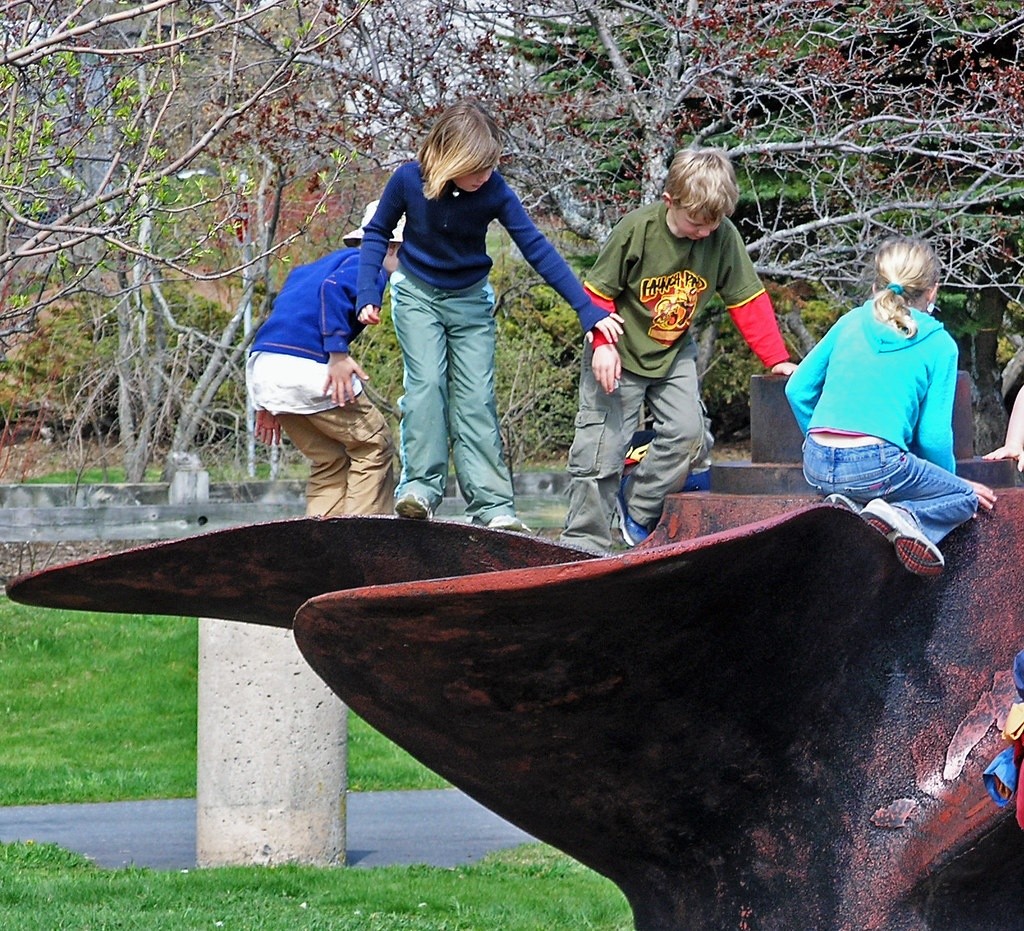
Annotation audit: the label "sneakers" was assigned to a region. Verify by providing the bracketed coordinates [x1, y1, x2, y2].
[616, 476, 648, 549]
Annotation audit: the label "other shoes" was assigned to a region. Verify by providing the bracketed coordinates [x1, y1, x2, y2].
[393, 493, 432, 522]
[821, 494, 863, 516]
[487, 516, 524, 532]
[858, 497, 945, 576]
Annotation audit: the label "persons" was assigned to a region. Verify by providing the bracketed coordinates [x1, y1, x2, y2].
[561, 147, 797, 553]
[357, 99, 624, 530]
[982, 385, 1024, 472]
[246, 200, 406, 516]
[786, 239, 997, 574]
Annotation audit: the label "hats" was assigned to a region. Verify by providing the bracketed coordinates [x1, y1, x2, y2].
[343, 199, 407, 248]
